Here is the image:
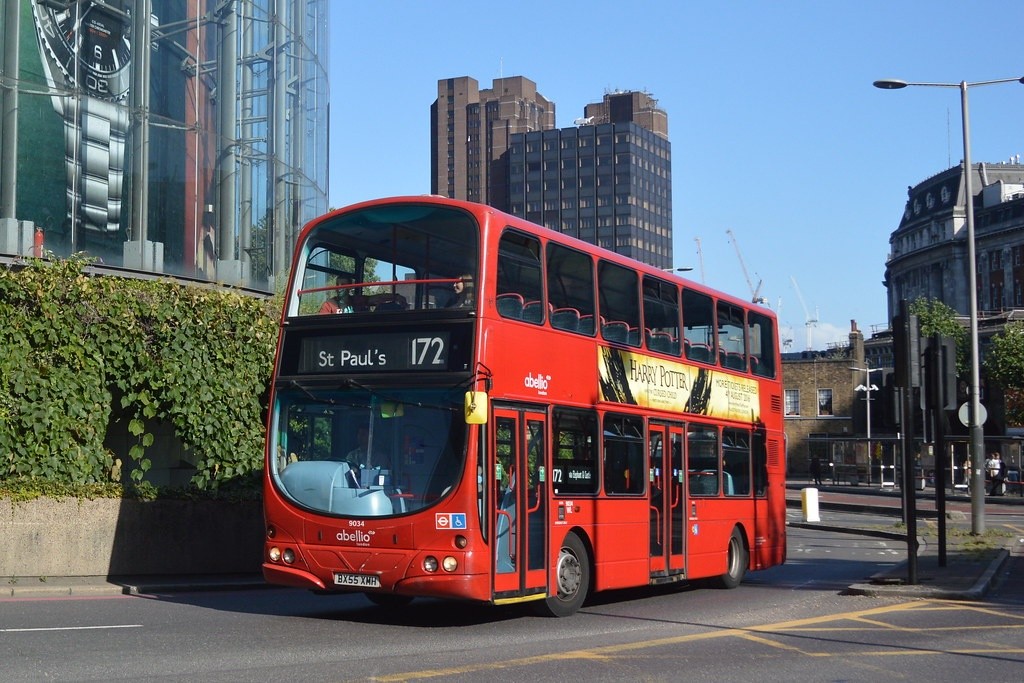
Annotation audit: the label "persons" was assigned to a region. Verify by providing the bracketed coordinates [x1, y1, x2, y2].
[317, 276, 354, 314]
[445, 273, 474, 308]
[345, 425, 390, 471]
[984, 452, 1005, 496]
[811, 453, 822, 485]
[961, 454, 972, 492]
[914, 452, 922, 467]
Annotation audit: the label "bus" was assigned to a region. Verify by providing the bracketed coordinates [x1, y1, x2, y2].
[261, 193, 786, 620]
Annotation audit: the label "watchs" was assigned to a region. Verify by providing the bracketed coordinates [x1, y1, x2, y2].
[31, 0, 160, 241]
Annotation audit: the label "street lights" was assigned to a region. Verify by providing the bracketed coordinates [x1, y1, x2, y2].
[655, 266, 695, 303]
[847, 367, 884, 484]
[728, 335, 741, 354]
[872, 76, 1024, 536]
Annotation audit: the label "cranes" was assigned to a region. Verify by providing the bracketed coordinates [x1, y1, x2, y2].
[693, 236, 704, 284]
[722, 228, 769, 305]
[790, 274, 821, 352]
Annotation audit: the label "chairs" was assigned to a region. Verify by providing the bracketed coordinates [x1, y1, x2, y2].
[700, 470, 734, 496]
[361, 291, 762, 373]
